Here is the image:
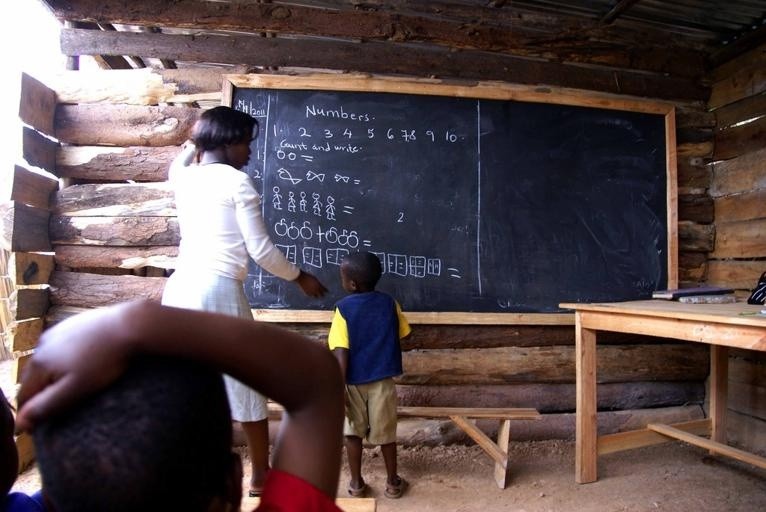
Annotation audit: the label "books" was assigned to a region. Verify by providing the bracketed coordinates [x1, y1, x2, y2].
[650, 285, 737, 301]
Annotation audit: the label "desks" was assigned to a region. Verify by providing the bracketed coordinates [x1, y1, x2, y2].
[559, 298, 766, 484]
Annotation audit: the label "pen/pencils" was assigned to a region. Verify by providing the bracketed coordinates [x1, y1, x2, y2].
[739, 311, 757, 316]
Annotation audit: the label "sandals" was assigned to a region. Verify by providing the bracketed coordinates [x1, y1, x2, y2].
[384, 477, 409, 498]
[347, 478, 367, 498]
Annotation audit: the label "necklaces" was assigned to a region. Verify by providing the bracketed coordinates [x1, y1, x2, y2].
[198, 161, 224, 164]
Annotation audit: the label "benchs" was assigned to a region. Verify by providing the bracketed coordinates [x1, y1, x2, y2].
[265, 403, 543, 489]
[241, 497, 377, 512]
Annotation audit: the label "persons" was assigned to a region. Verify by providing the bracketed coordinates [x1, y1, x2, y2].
[329, 250, 414, 500]
[0, 389, 49, 511]
[159, 105, 331, 498]
[14, 297, 346, 510]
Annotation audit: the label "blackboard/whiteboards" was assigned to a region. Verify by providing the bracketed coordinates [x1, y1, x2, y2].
[220, 73, 679, 325]
